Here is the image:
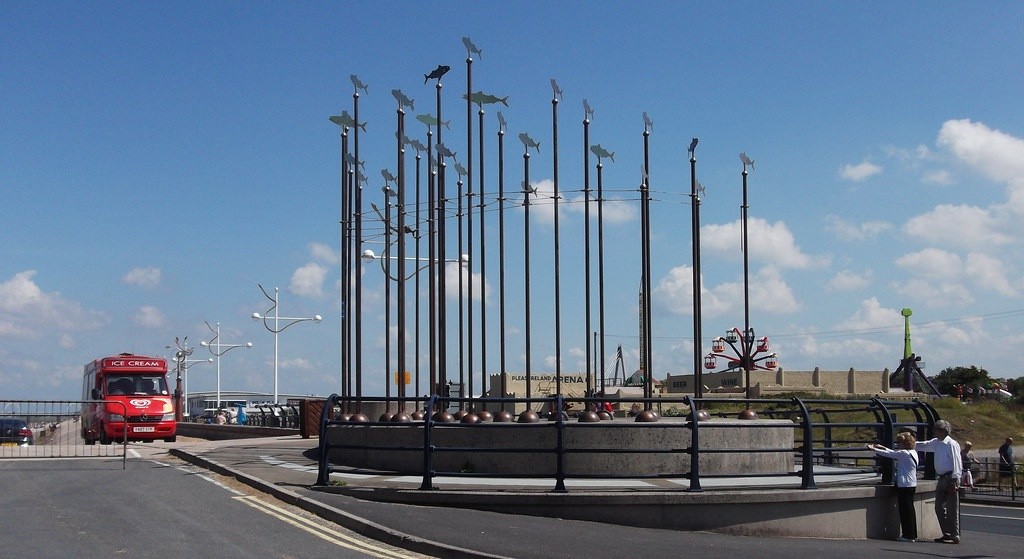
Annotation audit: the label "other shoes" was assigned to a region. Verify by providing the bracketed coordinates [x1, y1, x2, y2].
[997, 487, 1003, 491]
[934, 536, 960, 544]
[1016, 486, 1023, 491]
[971, 487, 979, 491]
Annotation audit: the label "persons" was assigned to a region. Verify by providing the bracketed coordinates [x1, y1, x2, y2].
[915, 419, 962, 543]
[960, 440, 983, 491]
[214, 411, 227, 425]
[867, 432, 918, 542]
[953, 383, 966, 401]
[997, 437, 1023, 491]
[229, 408, 238, 425]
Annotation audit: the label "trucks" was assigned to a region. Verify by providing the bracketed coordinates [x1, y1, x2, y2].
[80, 353, 180, 445]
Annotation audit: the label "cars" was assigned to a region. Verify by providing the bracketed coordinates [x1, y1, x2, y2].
[0, 420, 34, 446]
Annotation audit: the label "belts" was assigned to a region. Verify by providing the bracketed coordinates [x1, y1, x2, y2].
[937, 471, 953, 478]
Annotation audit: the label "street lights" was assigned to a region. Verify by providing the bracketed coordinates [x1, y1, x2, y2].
[250, 282, 322, 404]
[200, 320, 253, 416]
[163, 335, 213, 417]
[359, 250, 469, 398]
